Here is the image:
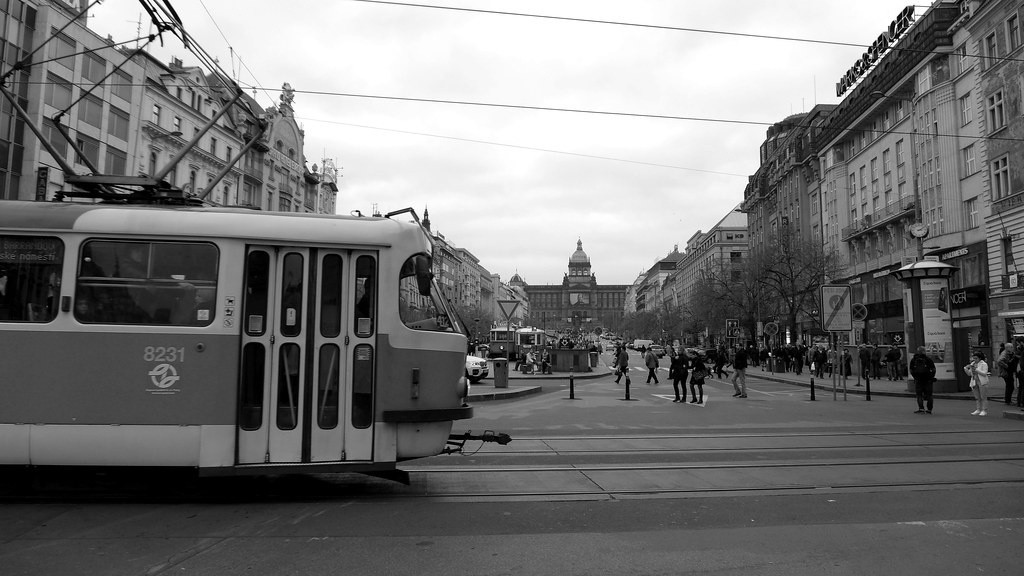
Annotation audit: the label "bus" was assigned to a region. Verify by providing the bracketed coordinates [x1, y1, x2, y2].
[515, 325, 550, 360]
[489, 324, 515, 361]
[0, 0, 511, 475]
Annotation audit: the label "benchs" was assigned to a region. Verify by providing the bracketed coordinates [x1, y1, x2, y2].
[28, 303, 48, 321]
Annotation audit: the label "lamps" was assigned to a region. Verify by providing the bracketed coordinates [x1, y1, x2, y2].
[911, 223, 927, 237]
[151, 131, 183, 139]
[869, 91, 912, 102]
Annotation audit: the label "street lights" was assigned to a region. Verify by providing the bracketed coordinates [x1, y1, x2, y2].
[870, 91, 928, 261]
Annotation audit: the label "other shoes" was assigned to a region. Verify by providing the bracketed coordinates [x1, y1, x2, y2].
[697, 400, 703, 404]
[925, 410, 931, 414]
[739, 394, 747, 398]
[615, 380, 619, 383]
[680, 397, 687, 402]
[512, 368, 518, 371]
[673, 397, 680, 402]
[691, 398, 697, 403]
[733, 392, 742, 397]
[914, 409, 925, 414]
[979, 411, 987, 416]
[971, 409, 980, 415]
[655, 381, 659, 384]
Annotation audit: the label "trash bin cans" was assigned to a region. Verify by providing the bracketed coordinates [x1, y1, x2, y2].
[590, 352, 598, 367]
[493, 358, 508, 388]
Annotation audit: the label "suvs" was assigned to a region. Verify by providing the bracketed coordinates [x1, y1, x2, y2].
[466, 355, 489, 383]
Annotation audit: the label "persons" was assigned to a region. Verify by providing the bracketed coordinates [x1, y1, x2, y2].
[859, 343, 905, 381]
[540, 349, 552, 374]
[356, 276, 371, 320]
[667, 344, 705, 404]
[748, 342, 827, 379]
[996, 336, 1024, 408]
[840, 348, 853, 379]
[31, 273, 56, 321]
[77, 286, 117, 322]
[526, 348, 541, 374]
[909, 346, 936, 414]
[546, 337, 602, 355]
[513, 351, 527, 371]
[468, 338, 479, 356]
[641, 345, 659, 384]
[713, 341, 737, 379]
[731, 343, 748, 398]
[963, 352, 989, 416]
[612, 344, 630, 385]
[939, 288, 946, 307]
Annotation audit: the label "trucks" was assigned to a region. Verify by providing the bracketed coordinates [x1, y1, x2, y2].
[634, 340, 657, 353]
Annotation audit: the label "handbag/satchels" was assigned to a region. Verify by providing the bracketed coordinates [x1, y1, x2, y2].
[692, 370, 708, 380]
[811, 362, 815, 370]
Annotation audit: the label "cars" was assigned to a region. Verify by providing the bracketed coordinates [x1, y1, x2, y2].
[650, 345, 663, 358]
[606, 344, 614, 351]
[600, 327, 634, 349]
[672, 346, 716, 363]
